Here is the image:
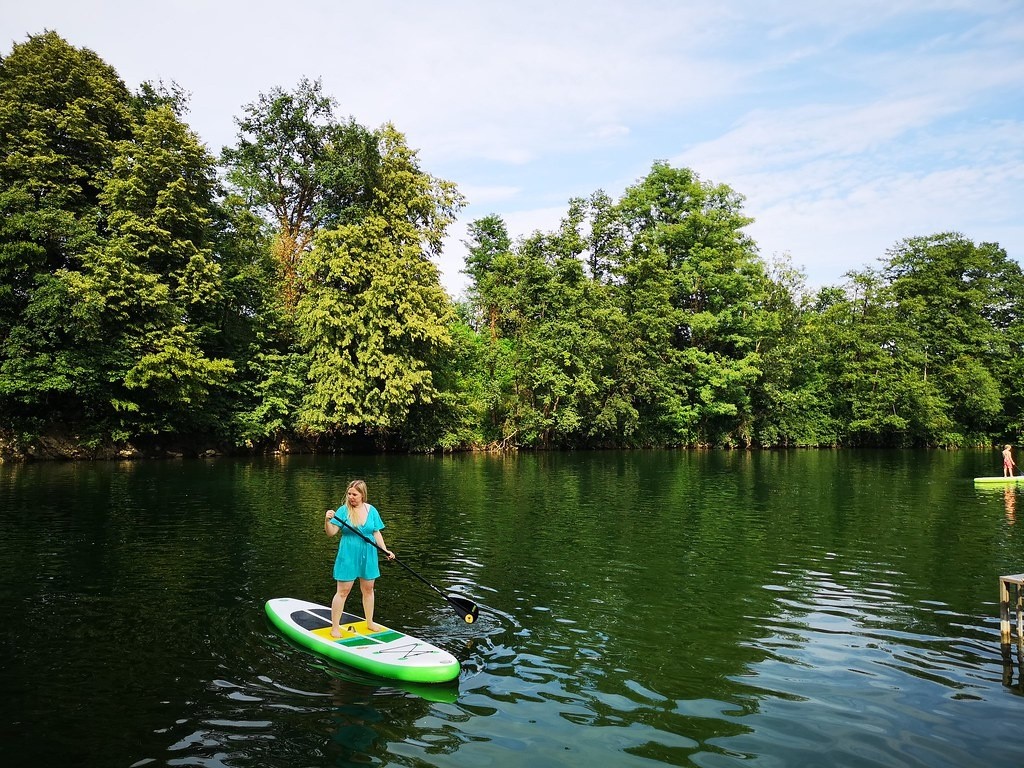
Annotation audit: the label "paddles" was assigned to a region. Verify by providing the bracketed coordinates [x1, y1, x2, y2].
[333, 516, 479, 624]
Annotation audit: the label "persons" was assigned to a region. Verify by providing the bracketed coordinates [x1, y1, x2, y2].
[324, 480, 395, 638]
[1002, 444, 1015, 477]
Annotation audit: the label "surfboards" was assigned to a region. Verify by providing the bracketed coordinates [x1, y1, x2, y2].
[264, 597, 461, 682]
